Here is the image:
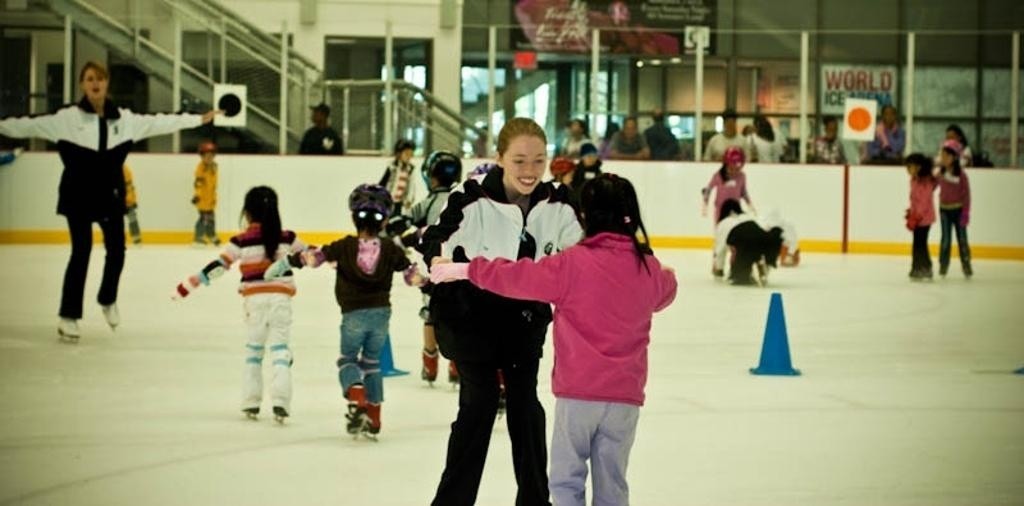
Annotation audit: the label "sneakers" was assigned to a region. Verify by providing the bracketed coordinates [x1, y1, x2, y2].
[909, 262, 972, 277]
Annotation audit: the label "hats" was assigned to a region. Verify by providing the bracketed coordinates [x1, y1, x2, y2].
[309, 102, 330, 111]
[580, 144, 596, 158]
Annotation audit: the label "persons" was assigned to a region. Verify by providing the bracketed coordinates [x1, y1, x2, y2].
[378, 139, 416, 238]
[414, 118, 585, 505]
[0, 60, 226, 341]
[905, 122, 973, 280]
[121, 166, 143, 246]
[701, 145, 785, 286]
[468, 163, 507, 420]
[172, 186, 321, 424]
[385, 149, 461, 388]
[557, 105, 680, 162]
[298, 102, 343, 155]
[701, 104, 905, 165]
[262, 183, 429, 442]
[430, 172, 678, 505]
[190, 142, 223, 248]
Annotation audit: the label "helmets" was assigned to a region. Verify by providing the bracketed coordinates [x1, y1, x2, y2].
[349, 185, 394, 227]
[550, 158, 575, 178]
[422, 149, 462, 193]
[199, 143, 218, 150]
[723, 145, 746, 168]
